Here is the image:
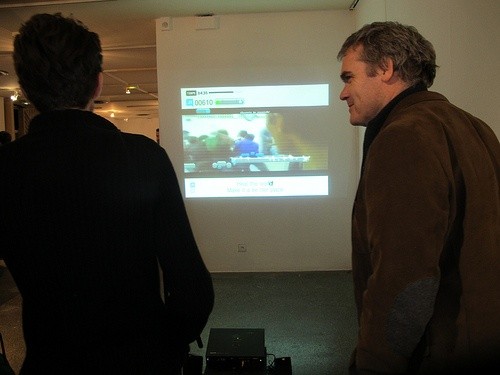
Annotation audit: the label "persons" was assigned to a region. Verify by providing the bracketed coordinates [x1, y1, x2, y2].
[338, 21, 500, 375]
[182, 109, 328, 170]
[156, 128, 160, 144]
[0, 130, 12, 145]
[0, 12, 214, 375]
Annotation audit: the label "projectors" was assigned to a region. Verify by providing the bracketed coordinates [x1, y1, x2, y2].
[206, 328, 266, 373]
